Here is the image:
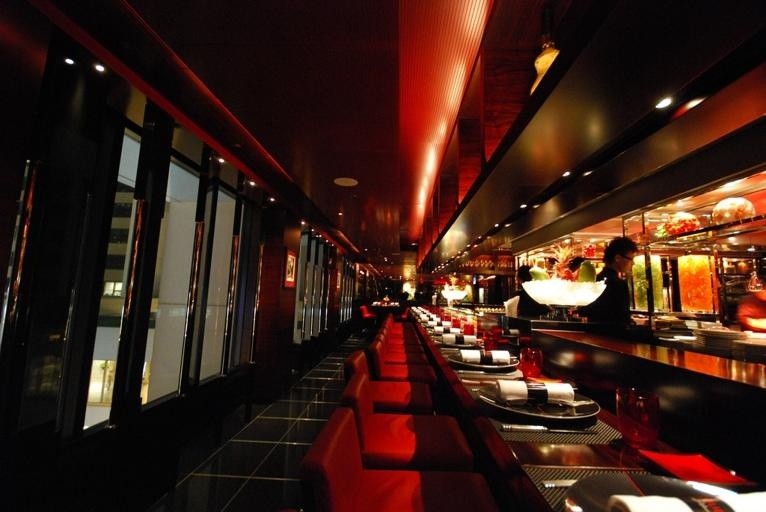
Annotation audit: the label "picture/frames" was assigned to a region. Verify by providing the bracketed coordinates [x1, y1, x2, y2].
[284, 250, 297, 290]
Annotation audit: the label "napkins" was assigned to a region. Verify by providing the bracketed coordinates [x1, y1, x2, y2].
[496, 380, 574, 407]
[406, 306, 508, 353]
[458, 350, 509, 363]
[609, 493, 765, 512]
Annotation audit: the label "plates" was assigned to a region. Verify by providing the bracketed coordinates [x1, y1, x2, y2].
[447, 353, 520, 370]
[564, 474, 735, 512]
[437, 338, 476, 349]
[477, 388, 601, 420]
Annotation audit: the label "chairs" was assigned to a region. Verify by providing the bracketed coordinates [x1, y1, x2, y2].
[368, 311, 433, 379]
[344, 352, 433, 414]
[307, 409, 492, 511]
[341, 374, 472, 469]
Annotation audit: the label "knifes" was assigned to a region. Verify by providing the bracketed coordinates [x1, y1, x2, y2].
[499, 424, 601, 435]
[458, 370, 517, 378]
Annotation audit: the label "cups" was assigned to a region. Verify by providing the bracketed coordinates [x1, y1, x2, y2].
[444, 312, 475, 335]
[520, 347, 543, 381]
[614, 386, 661, 450]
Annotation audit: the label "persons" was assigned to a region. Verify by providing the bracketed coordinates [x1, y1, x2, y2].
[487, 255, 585, 319]
[587, 236, 656, 339]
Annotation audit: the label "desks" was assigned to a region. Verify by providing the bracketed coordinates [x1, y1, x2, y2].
[508, 305, 765, 390]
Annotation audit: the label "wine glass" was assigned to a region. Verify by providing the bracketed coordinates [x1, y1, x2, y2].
[744, 258, 763, 293]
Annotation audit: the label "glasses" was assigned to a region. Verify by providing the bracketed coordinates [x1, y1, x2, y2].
[622, 254, 635, 263]
